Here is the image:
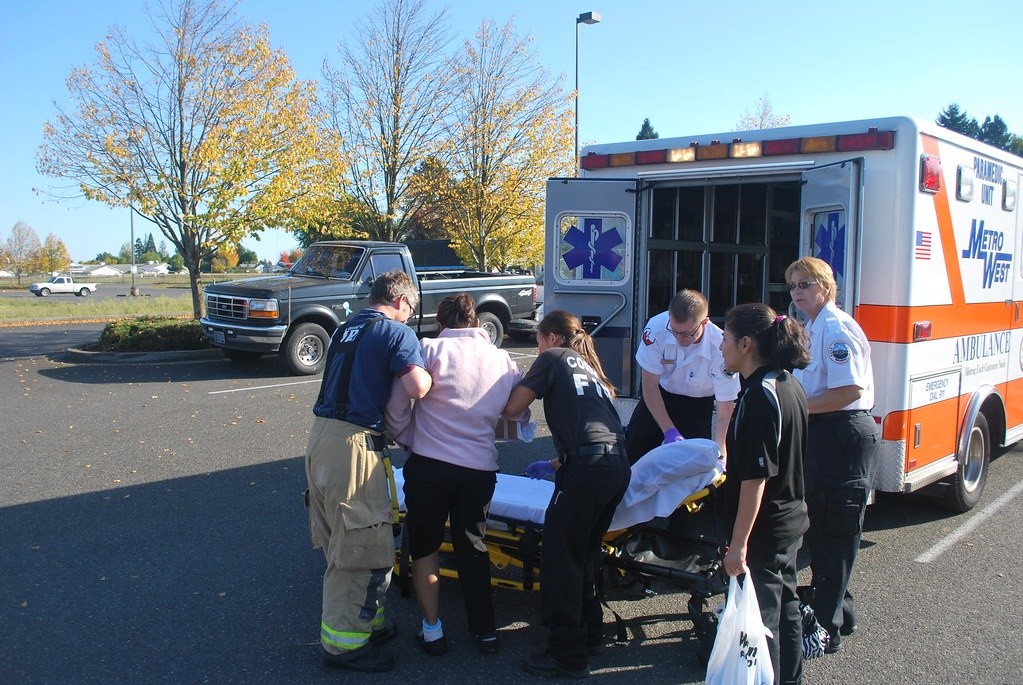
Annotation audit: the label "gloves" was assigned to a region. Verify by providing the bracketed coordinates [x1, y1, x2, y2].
[663, 429, 686, 444]
[525, 461, 555, 480]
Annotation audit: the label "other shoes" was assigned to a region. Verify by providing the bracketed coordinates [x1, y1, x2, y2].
[825, 642, 842, 652]
[840, 625, 858, 635]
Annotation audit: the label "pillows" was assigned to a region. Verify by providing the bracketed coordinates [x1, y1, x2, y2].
[624, 438, 719, 509]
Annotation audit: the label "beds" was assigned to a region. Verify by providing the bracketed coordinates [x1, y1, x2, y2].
[303, 437, 729, 600]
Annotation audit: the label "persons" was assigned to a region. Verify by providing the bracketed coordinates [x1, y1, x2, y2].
[385, 294, 531, 656]
[719, 304, 811, 685]
[506, 309, 632, 676]
[625, 289, 742, 472]
[302, 269, 433, 671]
[785, 257, 881, 649]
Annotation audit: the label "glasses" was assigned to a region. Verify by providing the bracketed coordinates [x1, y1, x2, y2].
[406, 299, 416, 321]
[787, 282, 818, 289]
[666, 319, 704, 338]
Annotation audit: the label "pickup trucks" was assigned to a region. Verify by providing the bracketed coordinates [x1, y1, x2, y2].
[30, 277, 98, 298]
[199, 240, 538, 376]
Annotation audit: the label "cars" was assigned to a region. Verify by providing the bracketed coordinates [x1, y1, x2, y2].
[500, 264, 546, 344]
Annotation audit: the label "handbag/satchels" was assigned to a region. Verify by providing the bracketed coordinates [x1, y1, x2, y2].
[705, 566, 775, 685]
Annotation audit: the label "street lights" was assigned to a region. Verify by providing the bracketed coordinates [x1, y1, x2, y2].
[573, 12, 602, 180]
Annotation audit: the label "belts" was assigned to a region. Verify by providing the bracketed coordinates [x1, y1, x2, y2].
[568, 446, 627, 458]
[809, 410, 866, 422]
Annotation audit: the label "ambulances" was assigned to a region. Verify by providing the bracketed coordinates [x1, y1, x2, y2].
[542, 116, 1023, 517]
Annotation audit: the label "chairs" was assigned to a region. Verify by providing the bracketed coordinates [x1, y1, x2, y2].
[344, 249, 364, 275]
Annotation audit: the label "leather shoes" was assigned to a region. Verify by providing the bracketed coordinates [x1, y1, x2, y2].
[470, 625, 500, 654]
[325, 645, 394, 672]
[369, 618, 397, 643]
[524, 649, 590, 677]
[416, 628, 447, 656]
[587, 633, 606, 655]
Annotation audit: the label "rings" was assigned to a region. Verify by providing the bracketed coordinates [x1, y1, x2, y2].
[736, 569, 739, 572]
